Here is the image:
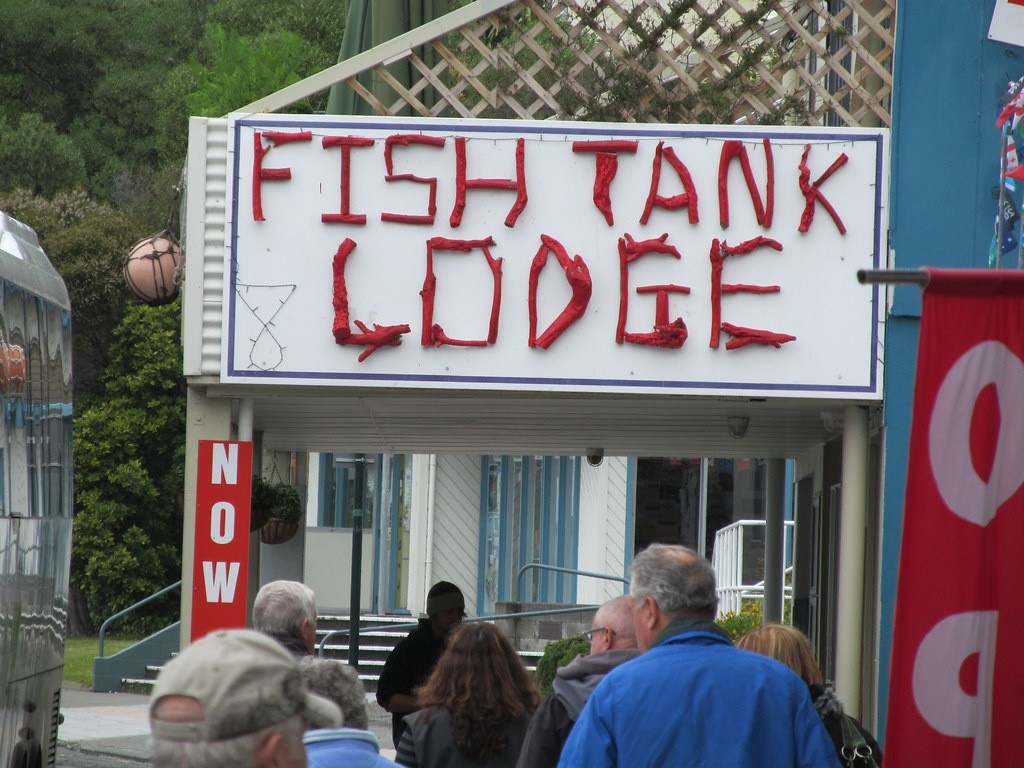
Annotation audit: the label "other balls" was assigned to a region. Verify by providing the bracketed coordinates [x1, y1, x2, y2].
[123, 237, 183, 303]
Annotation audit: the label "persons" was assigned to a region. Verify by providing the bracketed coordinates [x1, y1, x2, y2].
[251, 580, 318, 661]
[376, 581, 469, 749]
[150, 628, 345, 768]
[734, 625, 883, 768]
[555, 544, 843, 768]
[393, 622, 541, 768]
[517, 595, 642, 768]
[292, 655, 408, 768]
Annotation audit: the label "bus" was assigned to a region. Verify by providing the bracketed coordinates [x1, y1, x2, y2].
[0, 212, 75, 768]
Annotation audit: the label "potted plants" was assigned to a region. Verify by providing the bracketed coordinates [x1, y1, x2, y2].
[251, 474, 278, 532]
[260, 481, 304, 546]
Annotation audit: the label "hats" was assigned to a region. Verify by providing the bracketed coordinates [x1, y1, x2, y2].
[148, 628, 344, 742]
[427, 581, 465, 615]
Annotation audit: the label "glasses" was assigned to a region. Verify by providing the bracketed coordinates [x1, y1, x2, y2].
[585, 627, 617, 639]
